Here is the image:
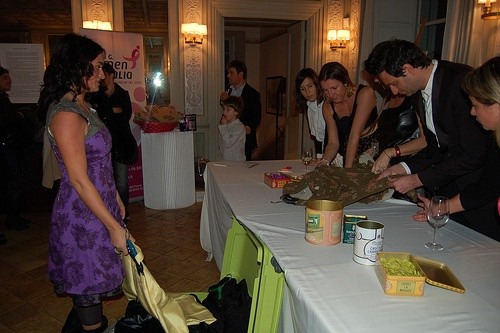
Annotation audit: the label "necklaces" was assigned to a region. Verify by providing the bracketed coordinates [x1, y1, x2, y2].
[73, 96, 96, 113]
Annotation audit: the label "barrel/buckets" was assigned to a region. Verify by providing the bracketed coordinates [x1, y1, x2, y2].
[304, 200, 343, 246]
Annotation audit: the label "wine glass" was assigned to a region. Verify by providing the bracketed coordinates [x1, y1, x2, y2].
[424, 196, 450, 251]
[301, 149, 313, 175]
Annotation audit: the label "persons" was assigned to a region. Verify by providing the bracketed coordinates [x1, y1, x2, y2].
[363, 39, 493, 228]
[216, 95, 246, 161]
[413, 56, 500, 241]
[315, 61, 378, 168]
[0, 67, 45, 245]
[86, 61, 139, 222]
[220, 59, 261, 161]
[295, 68, 326, 158]
[36, 33, 135, 333]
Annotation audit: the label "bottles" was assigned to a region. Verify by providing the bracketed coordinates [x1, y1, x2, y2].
[178, 111, 192, 132]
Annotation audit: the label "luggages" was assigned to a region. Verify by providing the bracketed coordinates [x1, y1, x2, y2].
[148, 217, 283, 333]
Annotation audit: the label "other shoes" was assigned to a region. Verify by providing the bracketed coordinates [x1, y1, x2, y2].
[6, 215, 33, 231]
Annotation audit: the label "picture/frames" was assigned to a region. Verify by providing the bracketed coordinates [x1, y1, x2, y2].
[265, 76, 282, 116]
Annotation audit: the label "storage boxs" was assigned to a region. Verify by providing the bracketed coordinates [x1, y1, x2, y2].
[264, 172, 290, 188]
[376, 250, 465, 296]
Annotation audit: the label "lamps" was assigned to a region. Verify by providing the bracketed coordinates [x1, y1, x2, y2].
[327, 30, 350, 50]
[478, 0, 500, 19]
[83, 20, 112, 31]
[180, 23, 207, 46]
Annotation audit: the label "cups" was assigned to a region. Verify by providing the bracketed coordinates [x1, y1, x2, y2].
[353, 221, 385, 266]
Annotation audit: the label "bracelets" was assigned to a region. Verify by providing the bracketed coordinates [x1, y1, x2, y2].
[394, 146, 400, 157]
[383, 151, 392, 158]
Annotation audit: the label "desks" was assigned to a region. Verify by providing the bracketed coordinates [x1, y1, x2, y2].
[140, 129, 196, 209]
[200, 156, 500, 333]
